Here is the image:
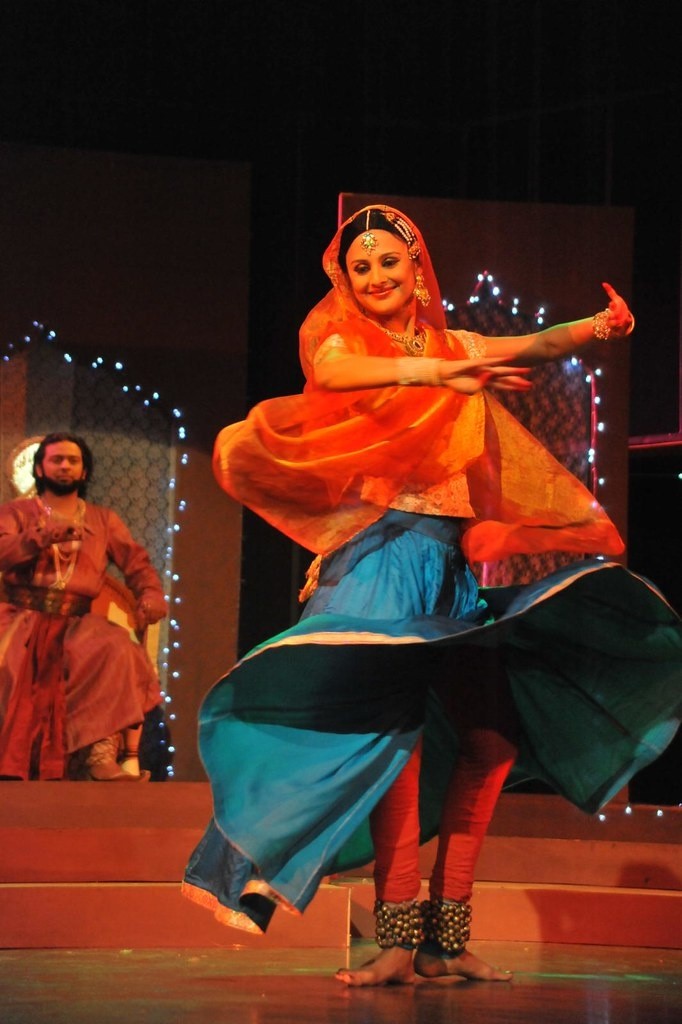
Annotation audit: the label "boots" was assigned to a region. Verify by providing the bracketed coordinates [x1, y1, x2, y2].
[68, 731, 150, 782]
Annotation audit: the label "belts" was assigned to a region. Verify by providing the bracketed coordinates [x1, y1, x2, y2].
[8, 583, 93, 617]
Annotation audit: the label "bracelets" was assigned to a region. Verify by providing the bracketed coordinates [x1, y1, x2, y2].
[593, 309, 635, 340]
[401, 357, 444, 386]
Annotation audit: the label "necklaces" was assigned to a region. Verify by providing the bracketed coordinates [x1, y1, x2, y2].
[43, 503, 83, 588]
[375, 323, 427, 357]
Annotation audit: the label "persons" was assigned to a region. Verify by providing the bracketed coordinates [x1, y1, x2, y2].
[182, 204, 682, 986]
[0, 433, 169, 784]
